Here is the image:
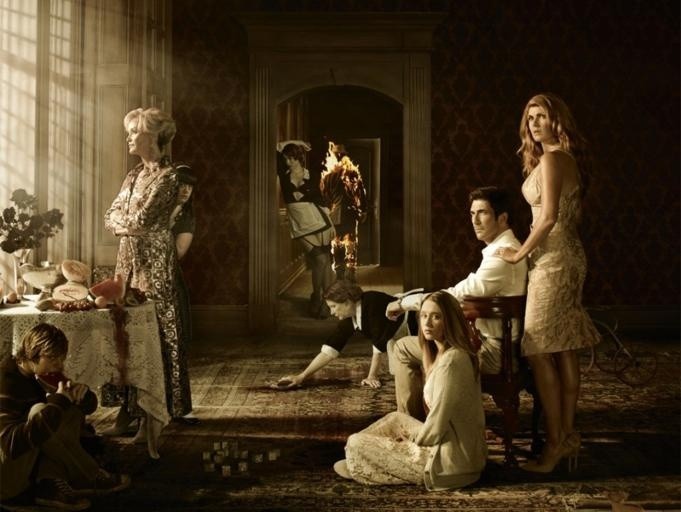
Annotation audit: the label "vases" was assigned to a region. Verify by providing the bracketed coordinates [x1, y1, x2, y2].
[12, 247, 37, 299]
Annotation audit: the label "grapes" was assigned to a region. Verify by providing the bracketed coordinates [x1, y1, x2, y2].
[53, 301, 92, 312]
[126, 287, 147, 304]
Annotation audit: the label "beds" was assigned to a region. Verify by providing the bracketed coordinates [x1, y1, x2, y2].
[1, 293, 162, 460]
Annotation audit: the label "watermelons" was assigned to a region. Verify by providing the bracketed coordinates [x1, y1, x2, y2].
[87, 273, 125, 304]
[35, 374, 64, 392]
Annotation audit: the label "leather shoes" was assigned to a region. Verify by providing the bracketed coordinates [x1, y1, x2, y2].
[310, 292, 330, 319]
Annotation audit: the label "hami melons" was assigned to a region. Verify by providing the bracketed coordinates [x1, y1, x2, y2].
[62, 260, 91, 282]
[51, 283, 87, 301]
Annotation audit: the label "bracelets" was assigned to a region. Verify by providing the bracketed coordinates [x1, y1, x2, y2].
[399, 296, 406, 311]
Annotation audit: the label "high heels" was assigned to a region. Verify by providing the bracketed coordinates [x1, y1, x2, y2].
[103, 405, 140, 436]
[134, 417, 162, 443]
[521, 432, 582, 473]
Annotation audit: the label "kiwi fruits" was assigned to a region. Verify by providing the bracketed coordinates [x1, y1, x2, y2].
[126, 297, 138, 305]
[35, 300, 52, 311]
[116, 296, 125, 307]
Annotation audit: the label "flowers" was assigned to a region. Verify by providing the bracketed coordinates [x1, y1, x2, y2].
[1, 185, 66, 256]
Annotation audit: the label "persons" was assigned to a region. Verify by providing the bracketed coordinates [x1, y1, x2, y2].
[276, 138, 339, 320]
[278, 278, 420, 389]
[385, 186, 530, 417]
[331, 289, 491, 492]
[101, 106, 195, 453]
[1, 322, 134, 512]
[500, 92, 604, 475]
[323, 141, 369, 285]
[170, 161, 200, 343]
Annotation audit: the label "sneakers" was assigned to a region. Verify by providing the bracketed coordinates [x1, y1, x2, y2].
[34, 478, 90, 510]
[71, 468, 131, 495]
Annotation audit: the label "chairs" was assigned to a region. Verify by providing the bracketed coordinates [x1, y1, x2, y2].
[459, 294, 549, 469]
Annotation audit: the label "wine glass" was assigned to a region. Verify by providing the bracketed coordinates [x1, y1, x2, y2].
[18, 263, 68, 302]
